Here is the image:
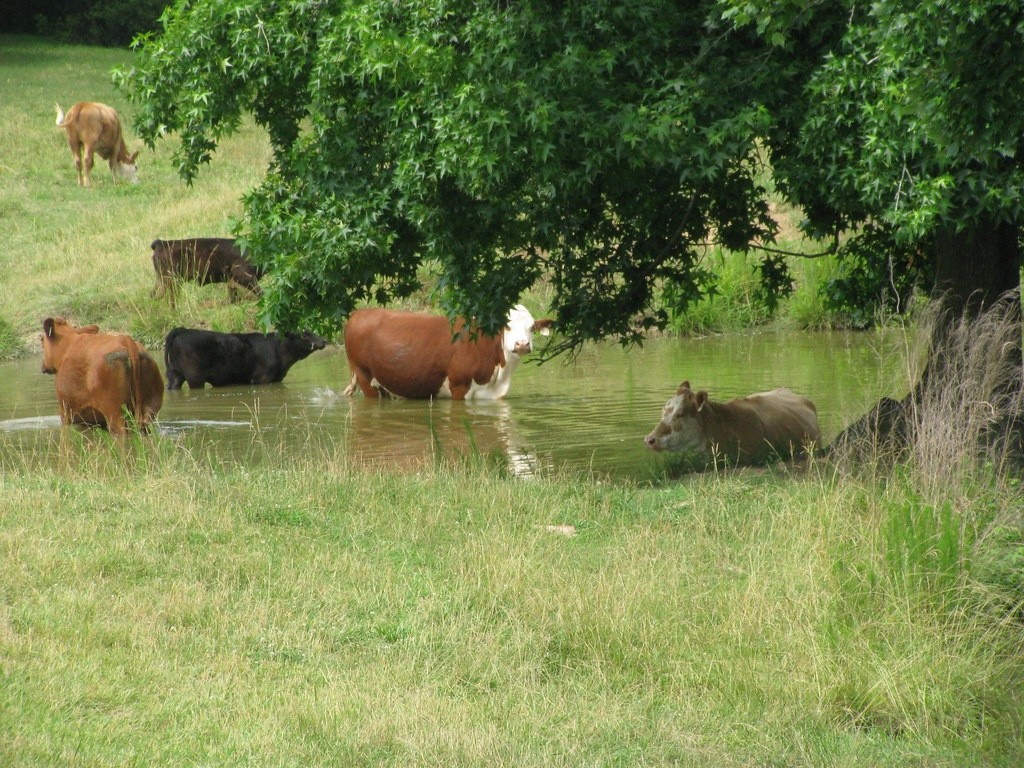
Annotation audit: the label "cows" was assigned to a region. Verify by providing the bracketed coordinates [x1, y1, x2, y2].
[53, 100, 139, 189]
[643, 380, 822, 466]
[163, 325, 326, 389]
[39, 317, 164, 435]
[338, 303, 552, 400]
[150, 237, 275, 310]
